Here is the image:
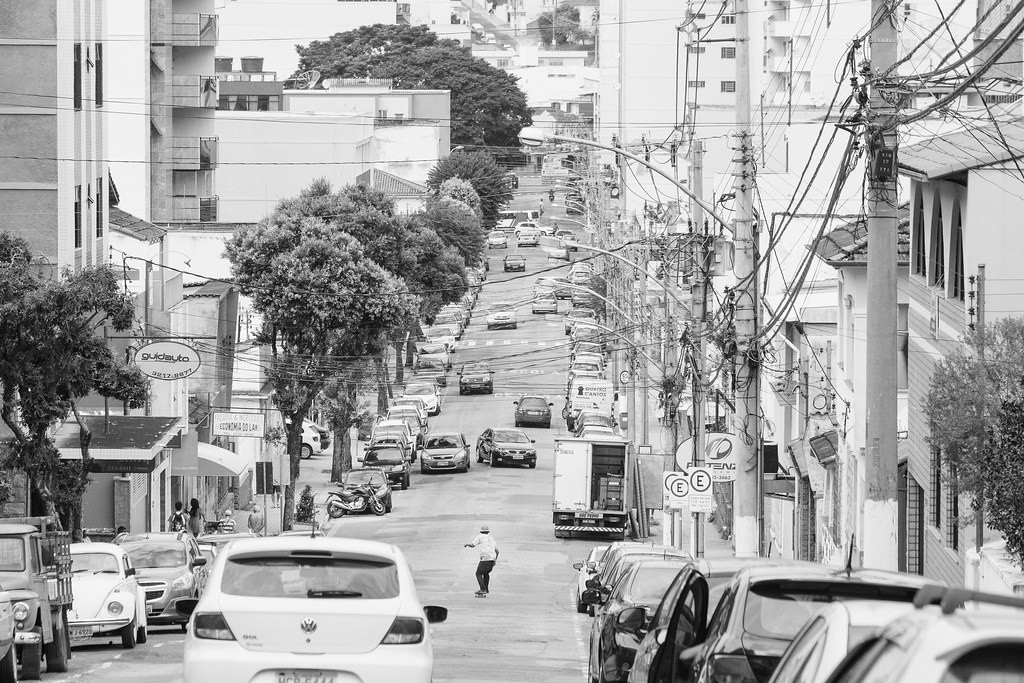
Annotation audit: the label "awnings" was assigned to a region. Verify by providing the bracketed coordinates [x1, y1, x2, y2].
[198, 441, 249, 476]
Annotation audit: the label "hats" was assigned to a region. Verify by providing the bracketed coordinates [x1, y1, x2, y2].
[480, 525, 490, 532]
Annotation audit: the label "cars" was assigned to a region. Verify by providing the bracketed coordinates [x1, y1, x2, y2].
[285, 416, 330, 459]
[356, 176, 618, 490]
[46, 542, 147, 648]
[477, 428, 536, 469]
[821, 585, 1024, 682]
[573, 541, 949, 683]
[766, 600, 962, 683]
[0, 586, 18, 683]
[184, 537, 448, 683]
[418, 431, 471, 474]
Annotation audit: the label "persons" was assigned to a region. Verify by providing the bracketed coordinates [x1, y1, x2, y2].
[83, 528, 91, 543]
[655, 392, 666, 426]
[271, 486, 280, 508]
[553, 223, 558, 236]
[540, 199, 544, 216]
[172, 498, 236, 534]
[549, 189, 554, 199]
[465, 527, 499, 594]
[248, 506, 265, 533]
[118, 526, 126, 533]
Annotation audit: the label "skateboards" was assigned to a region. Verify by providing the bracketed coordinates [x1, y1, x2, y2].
[475, 590, 489, 597]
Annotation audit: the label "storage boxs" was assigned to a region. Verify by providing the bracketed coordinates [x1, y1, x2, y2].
[600, 477, 624, 510]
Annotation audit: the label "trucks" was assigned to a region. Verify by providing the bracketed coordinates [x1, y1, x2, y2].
[0, 515, 73, 679]
[552, 439, 635, 542]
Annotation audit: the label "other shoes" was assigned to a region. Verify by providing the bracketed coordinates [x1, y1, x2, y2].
[475, 589, 489, 593]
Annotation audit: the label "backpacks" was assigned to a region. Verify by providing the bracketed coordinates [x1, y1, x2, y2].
[173, 513, 184, 531]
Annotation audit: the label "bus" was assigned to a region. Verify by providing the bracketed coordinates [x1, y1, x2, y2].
[492, 210, 541, 231]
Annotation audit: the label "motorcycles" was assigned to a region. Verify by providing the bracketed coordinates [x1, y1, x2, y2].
[325, 477, 386, 518]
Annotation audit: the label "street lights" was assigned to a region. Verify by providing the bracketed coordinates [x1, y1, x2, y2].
[538, 234, 707, 559]
[517, 127, 760, 560]
[544, 313, 682, 551]
[539, 276, 649, 445]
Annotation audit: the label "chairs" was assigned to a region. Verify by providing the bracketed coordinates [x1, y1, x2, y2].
[346, 572, 383, 594]
[238, 571, 283, 596]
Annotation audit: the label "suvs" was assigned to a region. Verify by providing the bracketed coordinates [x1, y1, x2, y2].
[196, 528, 263, 553]
[103, 529, 207, 632]
